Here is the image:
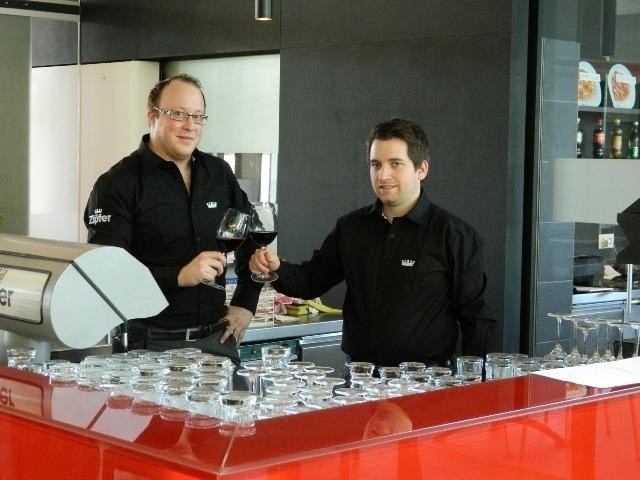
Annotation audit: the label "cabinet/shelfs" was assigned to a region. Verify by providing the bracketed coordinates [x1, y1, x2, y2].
[572, 291, 640, 358]
[237, 320, 352, 394]
[577, 56, 639, 160]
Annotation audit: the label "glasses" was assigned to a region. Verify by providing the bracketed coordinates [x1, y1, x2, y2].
[151, 105, 209, 125]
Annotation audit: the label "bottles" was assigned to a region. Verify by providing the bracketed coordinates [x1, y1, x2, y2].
[576, 116, 640, 159]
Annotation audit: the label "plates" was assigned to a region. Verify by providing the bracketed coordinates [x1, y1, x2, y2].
[578, 57, 637, 108]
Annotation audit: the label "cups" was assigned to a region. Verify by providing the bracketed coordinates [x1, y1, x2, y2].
[7, 312, 638, 438]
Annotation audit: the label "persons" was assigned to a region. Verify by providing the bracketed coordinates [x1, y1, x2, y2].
[84, 74, 267, 367]
[248, 118, 496, 390]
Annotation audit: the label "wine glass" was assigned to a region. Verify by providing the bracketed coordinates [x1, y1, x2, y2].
[198, 206, 250, 293]
[248, 201, 280, 284]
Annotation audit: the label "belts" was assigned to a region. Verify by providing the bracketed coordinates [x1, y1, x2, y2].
[120, 322, 231, 342]
